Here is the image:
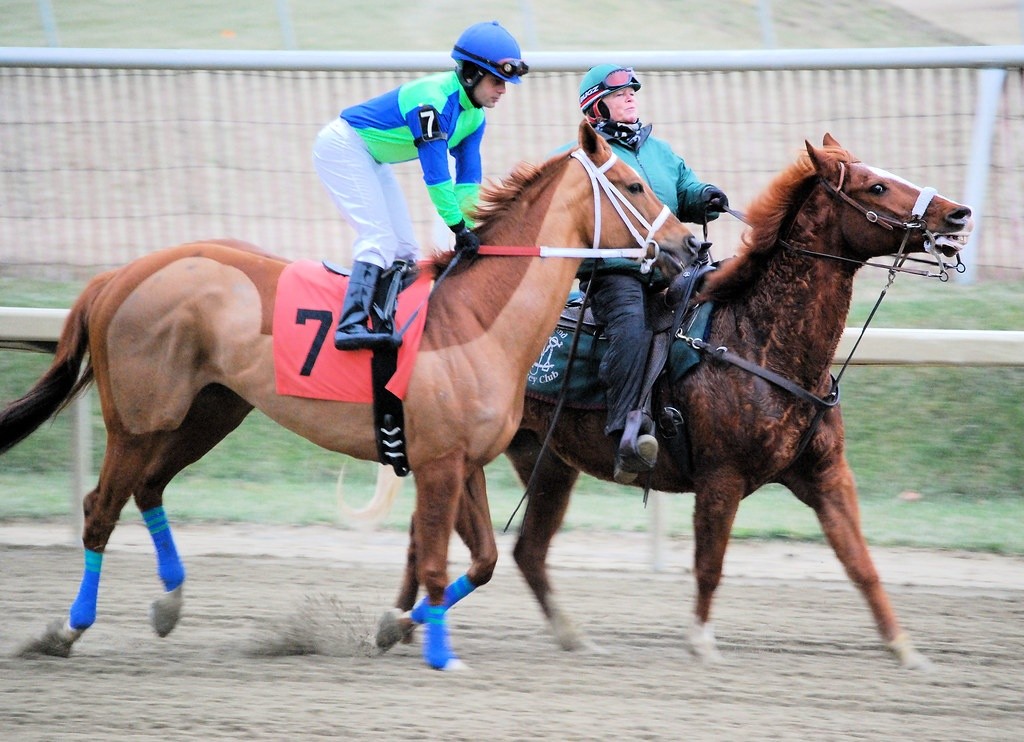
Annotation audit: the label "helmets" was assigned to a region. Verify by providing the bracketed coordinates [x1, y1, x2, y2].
[580, 64, 641, 112]
[449, 20, 526, 87]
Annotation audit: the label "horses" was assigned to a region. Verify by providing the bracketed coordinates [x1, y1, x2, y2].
[374, 132, 975, 673]
[0, 118, 703, 660]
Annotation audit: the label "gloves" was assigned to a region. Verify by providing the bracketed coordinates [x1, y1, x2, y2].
[453, 229, 479, 260]
[702, 188, 729, 213]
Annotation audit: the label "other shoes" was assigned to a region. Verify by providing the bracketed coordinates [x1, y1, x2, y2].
[612, 433, 657, 486]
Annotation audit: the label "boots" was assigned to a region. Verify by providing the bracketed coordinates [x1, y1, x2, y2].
[334, 259, 401, 350]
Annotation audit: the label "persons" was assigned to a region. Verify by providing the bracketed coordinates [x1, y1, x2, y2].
[312, 18, 530, 352]
[571, 62, 730, 482]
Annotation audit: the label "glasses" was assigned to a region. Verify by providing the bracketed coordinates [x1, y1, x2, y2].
[602, 68, 639, 88]
[499, 57, 529, 78]
